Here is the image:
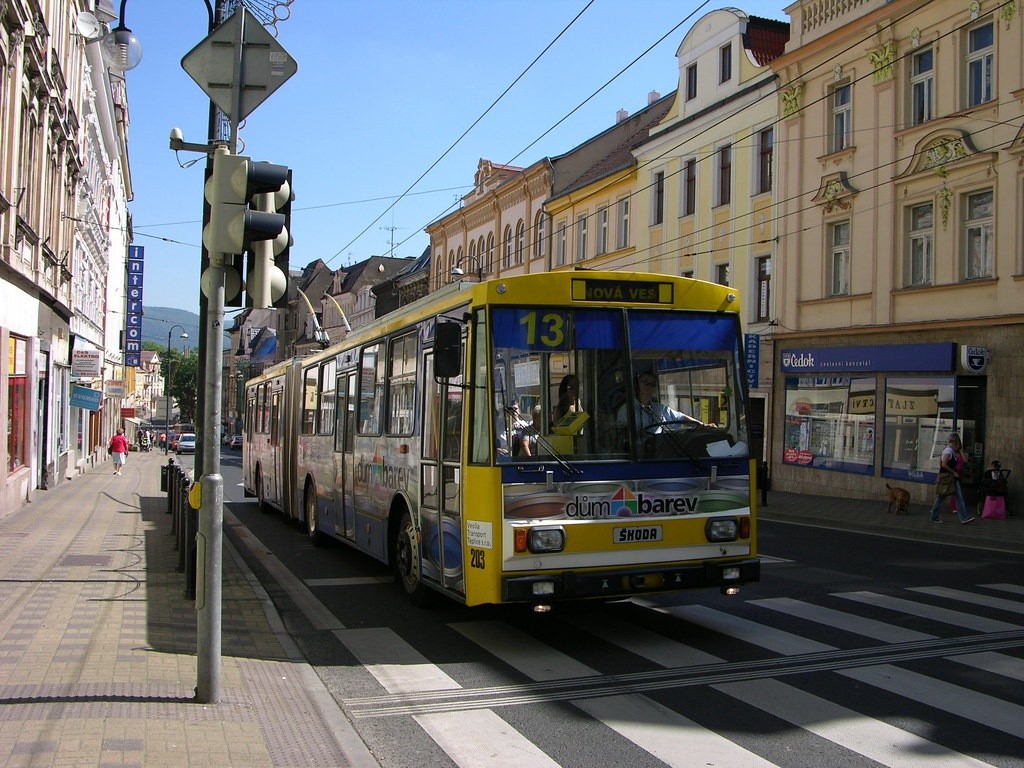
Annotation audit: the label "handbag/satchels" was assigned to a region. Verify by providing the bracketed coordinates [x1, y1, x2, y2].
[981, 496, 1007, 519]
[108, 445, 113, 454]
[936, 473, 955, 496]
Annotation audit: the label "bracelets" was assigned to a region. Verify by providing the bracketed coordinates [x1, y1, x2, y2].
[957, 446, 962, 451]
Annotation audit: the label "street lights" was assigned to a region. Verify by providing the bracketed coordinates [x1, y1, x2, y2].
[165, 325, 188, 455]
[451, 256, 482, 282]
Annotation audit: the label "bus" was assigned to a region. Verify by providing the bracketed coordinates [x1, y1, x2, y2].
[241, 267, 759, 613]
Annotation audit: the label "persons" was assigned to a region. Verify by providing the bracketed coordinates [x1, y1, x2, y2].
[138, 428, 177, 452]
[109, 428, 129, 476]
[552, 374, 580, 423]
[494, 400, 541, 456]
[930, 433, 976, 524]
[616, 372, 718, 452]
[991, 460, 1003, 480]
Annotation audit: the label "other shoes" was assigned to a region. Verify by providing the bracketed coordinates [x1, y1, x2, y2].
[930, 518, 943, 524]
[117, 473, 121, 475]
[114, 471, 117, 475]
[960, 517, 975, 524]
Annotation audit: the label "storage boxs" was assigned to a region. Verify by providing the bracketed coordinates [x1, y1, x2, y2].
[538, 411, 590, 456]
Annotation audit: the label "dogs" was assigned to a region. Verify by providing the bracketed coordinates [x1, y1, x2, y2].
[885, 483, 911, 516]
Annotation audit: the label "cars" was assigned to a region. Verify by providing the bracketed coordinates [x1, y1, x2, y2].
[222, 433, 243, 451]
[168, 432, 196, 455]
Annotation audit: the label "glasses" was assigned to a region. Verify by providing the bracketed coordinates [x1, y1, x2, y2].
[640, 381, 656, 387]
[567, 385, 574, 389]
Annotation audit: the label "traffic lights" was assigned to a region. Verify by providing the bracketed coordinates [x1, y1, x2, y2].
[212, 146, 288, 257]
